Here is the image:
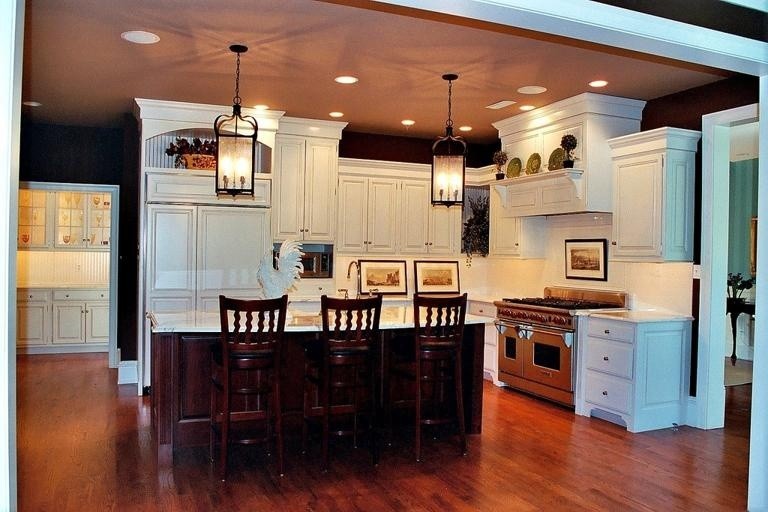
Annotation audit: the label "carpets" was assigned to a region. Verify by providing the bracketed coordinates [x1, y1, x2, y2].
[724, 358, 753, 386]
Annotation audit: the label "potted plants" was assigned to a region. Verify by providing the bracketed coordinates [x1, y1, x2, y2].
[559, 134, 577, 168]
[462, 196, 490, 268]
[727, 272, 754, 307]
[493, 151, 508, 180]
[164, 135, 218, 170]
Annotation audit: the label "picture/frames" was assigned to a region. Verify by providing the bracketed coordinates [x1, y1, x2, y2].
[414, 261, 460, 294]
[565, 240, 607, 281]
[358, 260, 407, 295]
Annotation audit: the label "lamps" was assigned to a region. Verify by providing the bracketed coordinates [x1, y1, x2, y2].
[213, 45, 258, 199]
[432, 74, 467, 207]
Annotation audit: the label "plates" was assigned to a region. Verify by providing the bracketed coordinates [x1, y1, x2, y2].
[507, 158, 521, 178]
[526, 154, 541, 174]
[549, 149, 565, 169]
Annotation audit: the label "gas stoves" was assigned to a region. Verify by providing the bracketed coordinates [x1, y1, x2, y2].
[493, 296, 621, 329]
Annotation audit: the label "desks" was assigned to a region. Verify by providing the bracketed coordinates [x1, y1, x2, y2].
[727, 305, 755, 366]
[146, 310, 496, 448]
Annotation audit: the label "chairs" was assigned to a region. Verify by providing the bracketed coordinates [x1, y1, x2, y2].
[301, 295, 383, 466]
[213, 296, 288, 480]
[388, 292, 468, 463]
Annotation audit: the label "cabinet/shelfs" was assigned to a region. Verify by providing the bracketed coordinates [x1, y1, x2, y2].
[490, 184, 547, 260]
[271, 114, 350, 246]
[53, 289, 109, 344]
[55, 192, 111, 249]
[16, 288, 53, 349]
[337, 158, 401, 257]
[463, 166, 489, 257]
[400, 164, 462, 256]
[130, 100, 283, 388]
[575, 308, 695, 434]
[603, 126, 703, 263]
[17, 189, 54, 251]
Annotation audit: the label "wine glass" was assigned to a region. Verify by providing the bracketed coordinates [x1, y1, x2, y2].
[22, 193, 100, 247]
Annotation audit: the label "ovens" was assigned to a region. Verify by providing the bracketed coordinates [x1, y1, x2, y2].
[494, 321, 574, 409]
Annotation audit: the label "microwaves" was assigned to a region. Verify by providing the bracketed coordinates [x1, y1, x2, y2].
[273, 243, 334, 279]
